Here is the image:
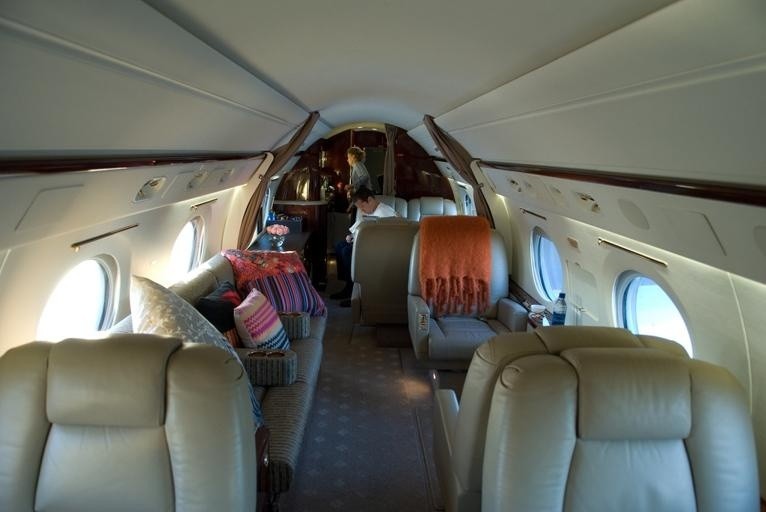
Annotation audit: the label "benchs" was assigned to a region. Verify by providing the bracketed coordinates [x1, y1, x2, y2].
[86, 245, 333, 501]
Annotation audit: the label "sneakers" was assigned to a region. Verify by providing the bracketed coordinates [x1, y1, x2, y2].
[339, 300, 351, 307]
[329, 289, 351, 299]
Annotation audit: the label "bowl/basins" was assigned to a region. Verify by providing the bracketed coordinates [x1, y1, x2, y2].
[530, 304, 545, 313]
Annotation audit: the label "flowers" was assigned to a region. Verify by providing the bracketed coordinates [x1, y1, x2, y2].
[266, 223, 287, 235]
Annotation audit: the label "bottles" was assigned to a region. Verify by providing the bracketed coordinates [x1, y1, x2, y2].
[551, 291, 568, 325]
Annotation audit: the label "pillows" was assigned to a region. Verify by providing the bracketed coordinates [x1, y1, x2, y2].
[225, 247, 328, 318]
[194, 276, 244, 347]
[124, 272, 265, 435]
[232, 291, 292, 354]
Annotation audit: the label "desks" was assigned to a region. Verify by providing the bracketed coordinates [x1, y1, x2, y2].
[249, 228, 312, 273]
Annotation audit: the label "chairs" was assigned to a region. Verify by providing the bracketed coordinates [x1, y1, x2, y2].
[408, 195, 463, 219]
[424, 324, 691, 511]
[0, 332, 262, 511]
[403, 214, 531, 372]
[476, 347, 765, 510]
[356, 194, 408, 223]
[349, 214, 417, 335]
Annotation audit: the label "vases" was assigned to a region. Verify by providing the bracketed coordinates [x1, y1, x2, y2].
[270, 235, 286, 246]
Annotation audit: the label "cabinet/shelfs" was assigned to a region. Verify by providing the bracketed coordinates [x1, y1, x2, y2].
[273, 198, 329, 289]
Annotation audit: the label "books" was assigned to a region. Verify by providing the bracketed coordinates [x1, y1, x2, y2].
[348, 215, 378, 234]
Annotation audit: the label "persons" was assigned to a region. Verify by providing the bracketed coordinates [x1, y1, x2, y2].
[328, 187, 400, 308]
[342, 145, 374, 213]
